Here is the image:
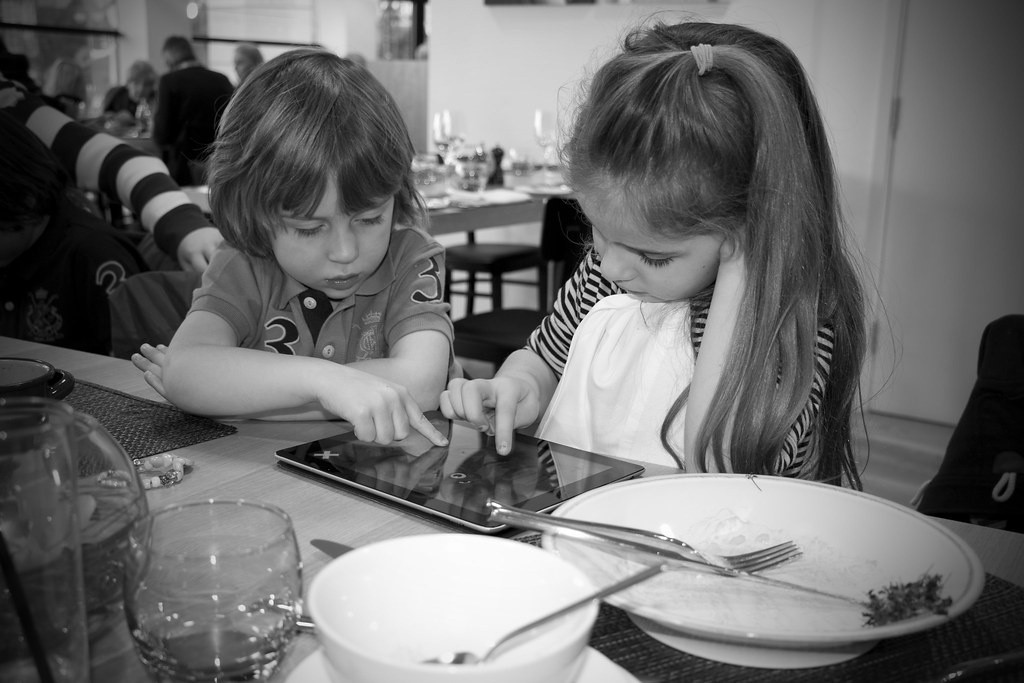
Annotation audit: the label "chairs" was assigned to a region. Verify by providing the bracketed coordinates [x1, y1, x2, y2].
[452, 196, 593, 381]
[443, 228, 547, 319]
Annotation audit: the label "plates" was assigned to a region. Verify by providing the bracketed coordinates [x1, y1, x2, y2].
[281, 645, 641, 683]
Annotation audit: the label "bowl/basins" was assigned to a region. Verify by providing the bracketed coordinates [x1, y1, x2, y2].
[540, 473, 985, 669]
[309, 533, 600, 683]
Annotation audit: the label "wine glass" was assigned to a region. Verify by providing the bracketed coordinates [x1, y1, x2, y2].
[533, 109, 564, 179]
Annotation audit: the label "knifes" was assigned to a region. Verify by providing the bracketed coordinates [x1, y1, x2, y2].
[310, 539, 354, 559]
[490, 509, 853, 603]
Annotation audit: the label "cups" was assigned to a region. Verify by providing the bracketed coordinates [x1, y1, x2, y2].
[76, 412, 150, 607]
[435, 111, 465, 167]
[455, 161, 488, 198]
[0, 397, 90, 683]
[412, 156, 450, 210]
[124, 498, 302, 683]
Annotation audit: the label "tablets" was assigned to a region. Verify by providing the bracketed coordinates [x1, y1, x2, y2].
[272, 410, 645, 538]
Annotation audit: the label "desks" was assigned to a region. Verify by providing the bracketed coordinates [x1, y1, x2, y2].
[0, 171, 1024, 683]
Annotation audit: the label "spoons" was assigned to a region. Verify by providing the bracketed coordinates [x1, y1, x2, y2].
[422, 561, 666, 666]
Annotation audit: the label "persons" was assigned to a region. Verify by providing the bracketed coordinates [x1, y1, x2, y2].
[0, 33, 266, 355]
[132, 49, 455, 447]
[440, 14, 867, 487]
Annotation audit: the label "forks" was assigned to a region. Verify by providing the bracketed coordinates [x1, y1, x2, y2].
[486, 496, 802, 577]
